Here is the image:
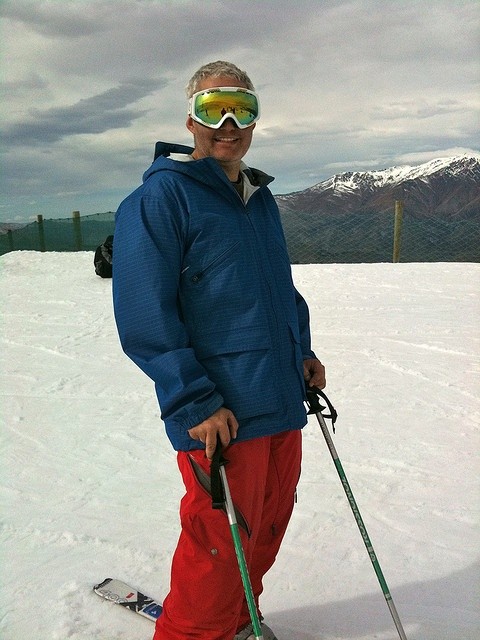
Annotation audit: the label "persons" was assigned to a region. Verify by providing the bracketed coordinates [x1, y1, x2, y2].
[110, 57, 330, 639]
[92, 234, 113, 281]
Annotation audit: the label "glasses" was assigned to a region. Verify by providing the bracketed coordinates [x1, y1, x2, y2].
[188, 87, 260, 130]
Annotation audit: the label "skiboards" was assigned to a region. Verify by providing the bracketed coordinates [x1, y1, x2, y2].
[93, 578, 163, 623]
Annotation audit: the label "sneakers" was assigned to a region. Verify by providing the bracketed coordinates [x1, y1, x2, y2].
[232, 621, 278, 640]
